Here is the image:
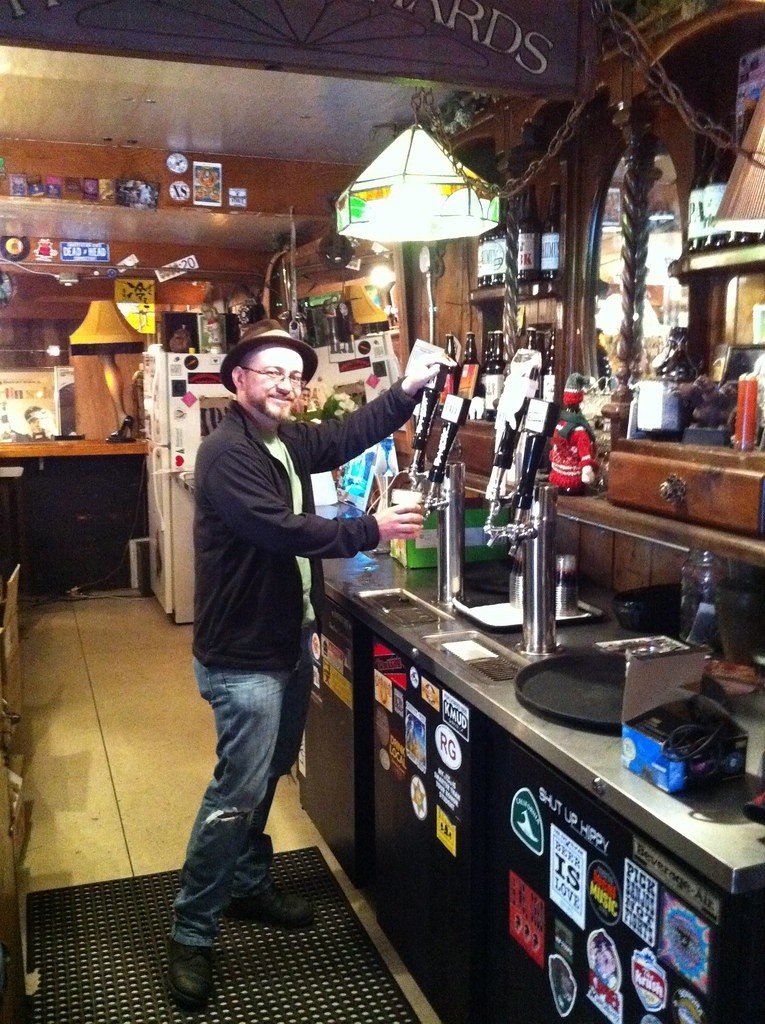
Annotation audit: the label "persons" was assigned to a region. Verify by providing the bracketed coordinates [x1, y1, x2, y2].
[336, 302, 353, 342]
[25, 406, 49, 440]
[120, 180, 152, 204]
[165, 320, 457, 1008]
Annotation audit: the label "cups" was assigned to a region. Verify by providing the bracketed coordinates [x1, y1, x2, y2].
[555, 554, 578, 617]
[390, 488, 423, 534]
[510, 547, 523, 609]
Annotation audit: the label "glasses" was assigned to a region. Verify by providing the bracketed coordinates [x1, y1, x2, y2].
[241, 365, 308, 393]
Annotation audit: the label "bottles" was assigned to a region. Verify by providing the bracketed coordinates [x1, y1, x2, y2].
[685, 118, 765, 253]
[436, 328, 554, 423]
[478, 180, 561, 287]
[680, 553, 727, 657]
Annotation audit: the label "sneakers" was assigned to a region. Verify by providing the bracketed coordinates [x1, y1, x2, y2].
[224, 880, 315, 930]
[166, 935, 212, 1010]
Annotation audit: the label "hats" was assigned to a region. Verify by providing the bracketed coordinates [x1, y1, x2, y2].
[219, 317, 319, 395]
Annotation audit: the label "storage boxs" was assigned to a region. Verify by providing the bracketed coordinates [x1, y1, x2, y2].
[387, 494, 514, 567]
[0, 563, 21, 733]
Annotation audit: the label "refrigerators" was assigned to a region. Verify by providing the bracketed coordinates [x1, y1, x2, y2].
[144, 350, 237, 623]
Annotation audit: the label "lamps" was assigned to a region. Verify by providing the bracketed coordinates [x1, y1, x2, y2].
[334, 105, 500, 241]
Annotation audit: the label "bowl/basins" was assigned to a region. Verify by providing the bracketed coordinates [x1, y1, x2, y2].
[611, 584, 679, 632]
